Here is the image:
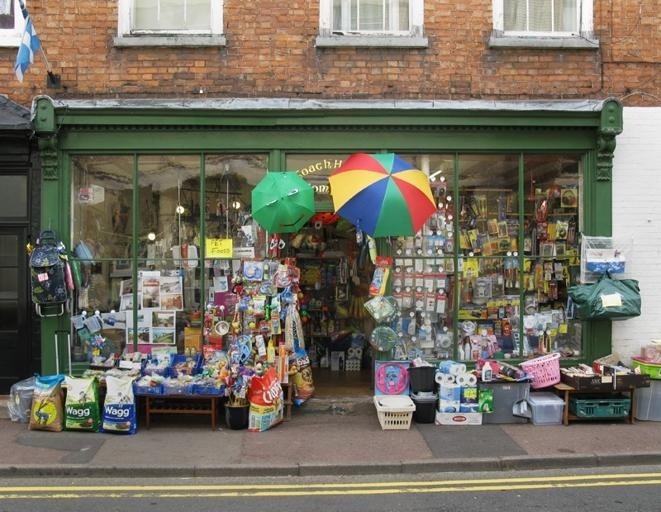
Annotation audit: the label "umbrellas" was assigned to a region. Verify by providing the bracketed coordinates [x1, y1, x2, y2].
[251, 171, 316, 235]
[329, 151, 436, 239]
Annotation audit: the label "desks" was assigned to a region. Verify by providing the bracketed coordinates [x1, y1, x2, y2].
[144, 393, 220, 431]
[553, 380, 638, 426]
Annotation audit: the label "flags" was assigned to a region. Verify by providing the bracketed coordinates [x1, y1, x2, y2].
[14, 15, 42, 84]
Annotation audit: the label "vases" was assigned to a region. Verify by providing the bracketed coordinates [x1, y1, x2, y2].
[225, 403, 248, 430]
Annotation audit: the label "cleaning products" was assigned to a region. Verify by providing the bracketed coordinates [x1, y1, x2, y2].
[481, 361, 493, 382]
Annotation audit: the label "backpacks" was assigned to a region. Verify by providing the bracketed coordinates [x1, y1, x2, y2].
[28, 229, 69, 306]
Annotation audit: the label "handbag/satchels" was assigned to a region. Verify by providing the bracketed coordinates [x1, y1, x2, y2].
[566, 270, 642, 320]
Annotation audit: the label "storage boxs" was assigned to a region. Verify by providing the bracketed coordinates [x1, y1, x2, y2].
[528, 391, 568, 427]
[632, 377, 660, 422]
[475, 376, 534, 426]
[412, 392, 437, 424]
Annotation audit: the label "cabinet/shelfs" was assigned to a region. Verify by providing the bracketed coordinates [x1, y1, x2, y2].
[293, 254, 347, 340]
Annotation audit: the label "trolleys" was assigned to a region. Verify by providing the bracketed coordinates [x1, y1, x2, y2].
[27, 228, 76, 318]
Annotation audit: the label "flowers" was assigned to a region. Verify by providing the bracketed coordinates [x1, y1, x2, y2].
[224, 383, 249, 406]
